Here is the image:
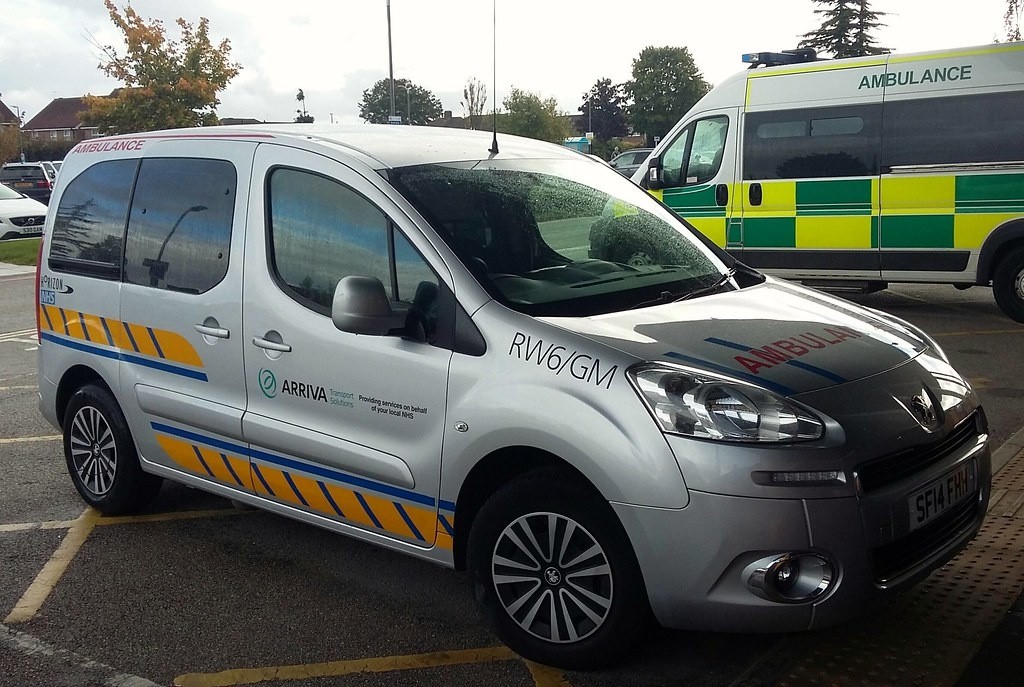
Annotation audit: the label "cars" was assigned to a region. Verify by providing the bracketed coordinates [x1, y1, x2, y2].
[42, 161, 58, 182]
[52, 161, 63, 172]
[0, 182, 48, 240]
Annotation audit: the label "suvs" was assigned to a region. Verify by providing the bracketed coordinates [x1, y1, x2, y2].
[606, 148, 701, 179]
[0, 162, 53, 206]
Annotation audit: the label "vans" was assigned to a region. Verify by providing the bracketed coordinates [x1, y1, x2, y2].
[38, 122, 993, 671]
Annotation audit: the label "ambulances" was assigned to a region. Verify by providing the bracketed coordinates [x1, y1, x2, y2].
[587, 42, 1024, 324]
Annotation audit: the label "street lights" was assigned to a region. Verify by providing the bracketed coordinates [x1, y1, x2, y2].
[13, 106, 25, 162]
[150, 205, 209, 288]
